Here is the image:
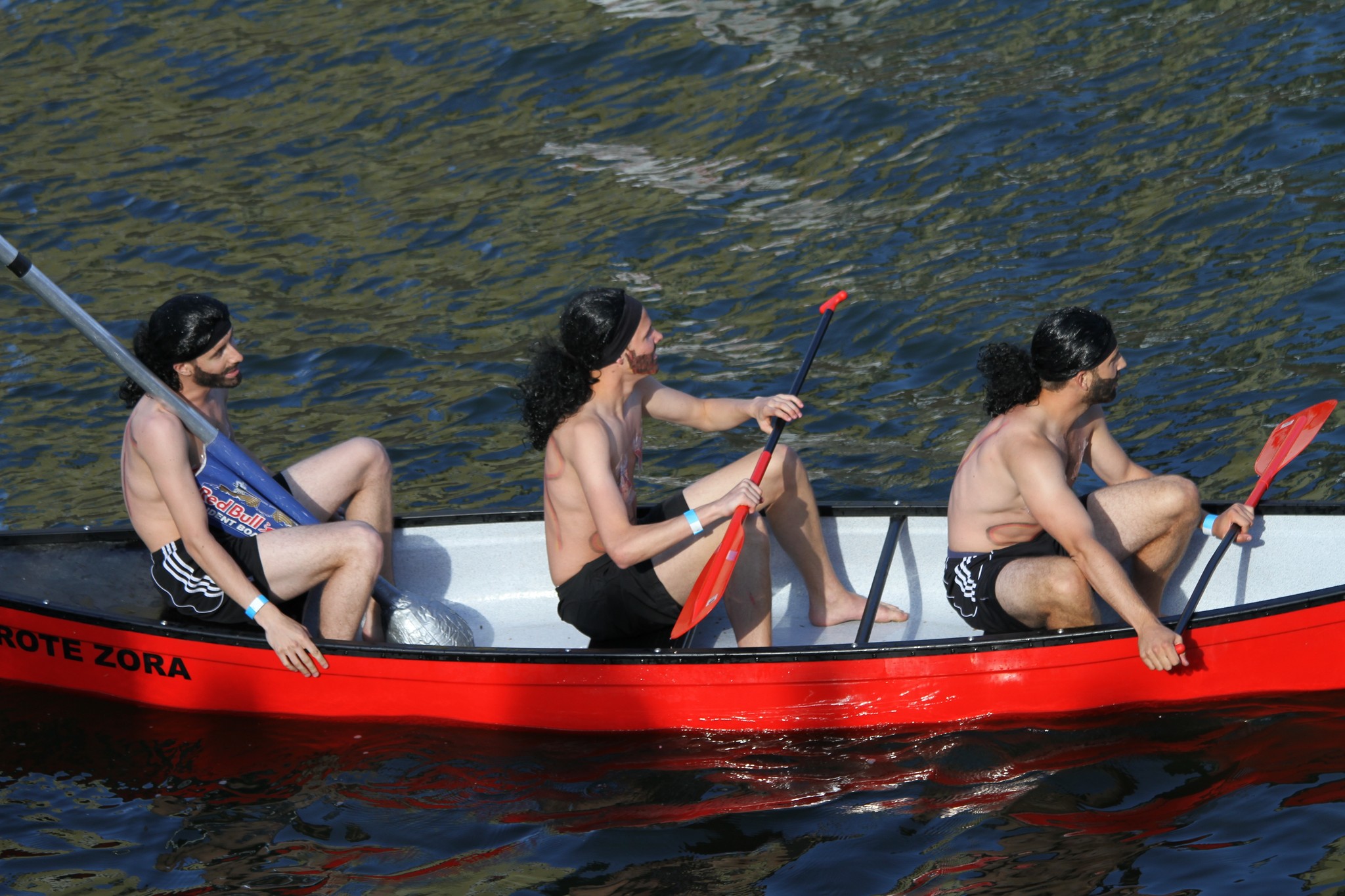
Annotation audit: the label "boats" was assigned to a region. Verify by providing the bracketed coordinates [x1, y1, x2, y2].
[0, 501, 1345, 737]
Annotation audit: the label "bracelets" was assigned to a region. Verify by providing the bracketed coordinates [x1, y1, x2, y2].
[683, 509, 704, 536]
[1202, 513, 1218, 537]
[244, 594, 269, 620]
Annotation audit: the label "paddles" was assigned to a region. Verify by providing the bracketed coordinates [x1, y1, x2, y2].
[668, 287, 850, 640]
[1170, 400, 1337, 659]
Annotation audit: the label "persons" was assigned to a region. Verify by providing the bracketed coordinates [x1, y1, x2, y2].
[507, 288, 909, 648]
[942, 305, 1254, 671]
[119, 293, 395, 677]
